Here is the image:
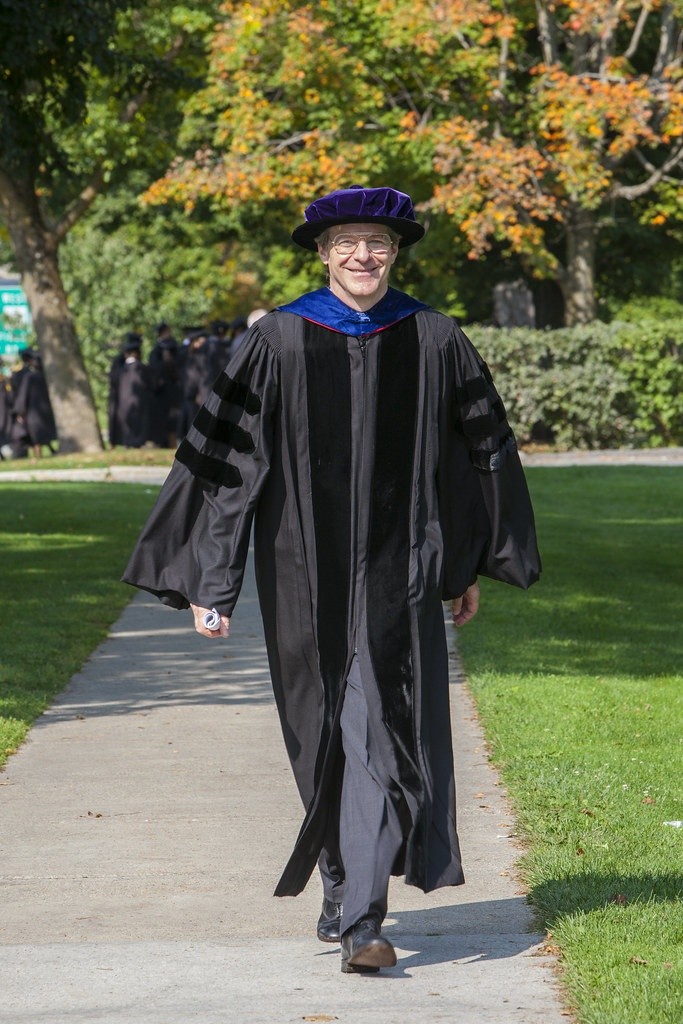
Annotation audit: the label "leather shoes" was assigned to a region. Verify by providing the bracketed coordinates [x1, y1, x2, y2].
[317, 892, 397, 973]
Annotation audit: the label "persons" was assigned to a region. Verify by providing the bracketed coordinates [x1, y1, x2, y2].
[1, 308, 269, 458]
[121, 186, 544, 974]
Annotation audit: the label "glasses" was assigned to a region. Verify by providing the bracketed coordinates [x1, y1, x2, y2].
[331, 233, 392, 254]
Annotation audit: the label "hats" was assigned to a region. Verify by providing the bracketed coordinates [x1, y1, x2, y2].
[292, 185, 425, 252]
